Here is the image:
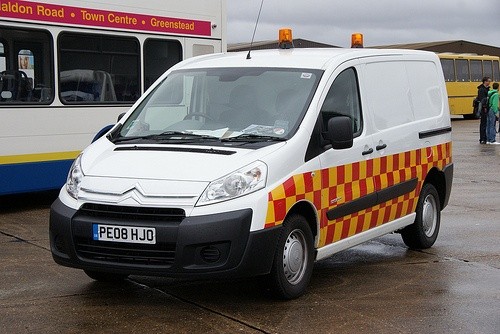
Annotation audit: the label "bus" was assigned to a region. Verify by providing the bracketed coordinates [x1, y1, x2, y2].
[437, 52, 500, 119]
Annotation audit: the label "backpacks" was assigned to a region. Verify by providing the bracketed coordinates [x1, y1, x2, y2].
[477, 91, 498, 117]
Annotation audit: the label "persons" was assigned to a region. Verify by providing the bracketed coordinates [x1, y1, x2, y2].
[477, 77, 491, 144]
[485, 82, 500, 146]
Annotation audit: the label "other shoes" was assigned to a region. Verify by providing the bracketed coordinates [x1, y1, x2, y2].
[490, 142, 500, 144]
[486, 142, 490, 145]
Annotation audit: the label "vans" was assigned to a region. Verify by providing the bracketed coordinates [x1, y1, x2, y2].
[0, 0, 454, 300]
